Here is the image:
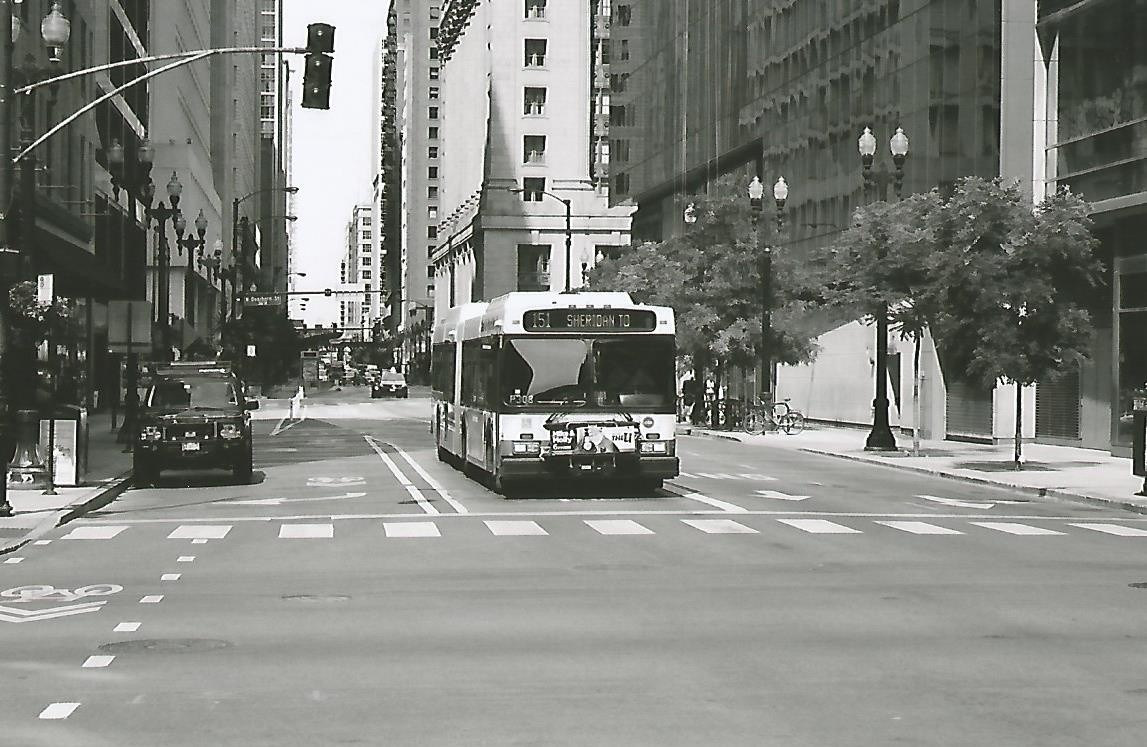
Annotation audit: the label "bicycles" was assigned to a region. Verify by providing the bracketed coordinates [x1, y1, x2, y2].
[702, 396, 805, 436]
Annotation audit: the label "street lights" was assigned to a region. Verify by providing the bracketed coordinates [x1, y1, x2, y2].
[859, 126, 910, 451]
[241, 215, 298, 313]
[10, 0, 73, 491]
[214, 255, 236, 340]
[683, 206, 716, 425]
[174, 207, 209, 359]
[142, 170, 183, 360]
[231, 187, 300, 321]
[749, 175, 789, 426]
[196, 238, 225, 360]
[107, 137, 155, 444]
[272, 272, 307, 313]
[580, 248, 605, 288]
[508, 186, 571, 292]
[397, 299, 430, 386]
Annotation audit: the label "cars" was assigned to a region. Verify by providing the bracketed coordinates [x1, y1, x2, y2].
[319, 365, 408, 399]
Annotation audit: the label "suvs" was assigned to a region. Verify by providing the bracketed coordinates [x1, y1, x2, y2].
[133, 361, 259, 487]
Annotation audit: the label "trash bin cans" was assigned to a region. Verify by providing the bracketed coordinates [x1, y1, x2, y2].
[39, 403, 88, 487]
[1130, 389, 1147, 497]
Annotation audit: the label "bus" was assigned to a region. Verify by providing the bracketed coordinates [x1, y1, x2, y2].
[429, 291, 695, 497]
